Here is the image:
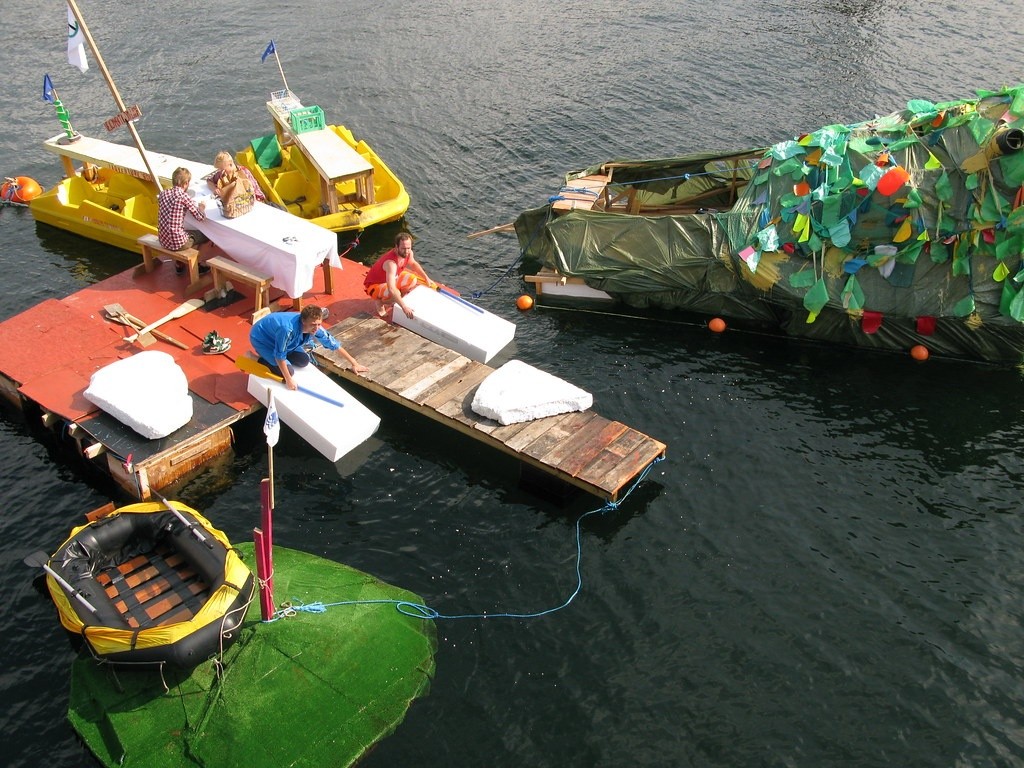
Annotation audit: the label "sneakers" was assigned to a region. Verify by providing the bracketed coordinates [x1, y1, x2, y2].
[202, 335, 230, 354]
[204, 330, 232, 344]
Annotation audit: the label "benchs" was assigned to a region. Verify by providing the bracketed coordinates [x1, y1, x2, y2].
[204, 255, 282, 326]
[138, 233, 213, 297]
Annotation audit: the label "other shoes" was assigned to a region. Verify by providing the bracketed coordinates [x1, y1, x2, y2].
[197, 263, 211, 275]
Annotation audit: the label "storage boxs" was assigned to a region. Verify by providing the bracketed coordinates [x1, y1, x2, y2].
[271, 90, 300, 113]
[290, 105, 325, 133]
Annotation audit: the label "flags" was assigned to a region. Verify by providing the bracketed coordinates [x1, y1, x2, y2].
[67, 4, 90, 74]
[262, 40, 276, 63]
[43, 73, 54, 104]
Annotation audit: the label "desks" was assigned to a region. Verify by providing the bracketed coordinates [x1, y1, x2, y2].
[266, 98, 374, 213]
[157, 179, 344, 312]
[42, 131, 218, 191]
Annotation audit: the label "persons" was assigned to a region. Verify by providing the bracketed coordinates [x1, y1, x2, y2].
[239, 304, 370, 391]
[363, 232, 437, 320]
[157, 168, 211, 276]
[208, 152, 267, 204]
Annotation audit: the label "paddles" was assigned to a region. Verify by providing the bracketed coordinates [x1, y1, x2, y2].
[416, 277, 484, 313]
[103, 298, 205, 350]
[235, 354, 344, 408]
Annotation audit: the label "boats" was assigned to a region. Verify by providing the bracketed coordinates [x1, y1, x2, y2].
[521, 80, 1024, 377]
[234, 87, 411, 234]
[27, 71, 222, 258]
[42, 496, 256, 675]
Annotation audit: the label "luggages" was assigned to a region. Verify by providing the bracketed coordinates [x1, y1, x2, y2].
[174, 264, 186, 275]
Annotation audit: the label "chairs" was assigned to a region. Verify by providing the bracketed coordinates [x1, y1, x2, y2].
[55, 174, 160, 229]
[248, 124, 379, 218]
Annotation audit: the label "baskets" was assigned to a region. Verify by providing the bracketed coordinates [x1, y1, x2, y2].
[217, 194, 254, 218]
